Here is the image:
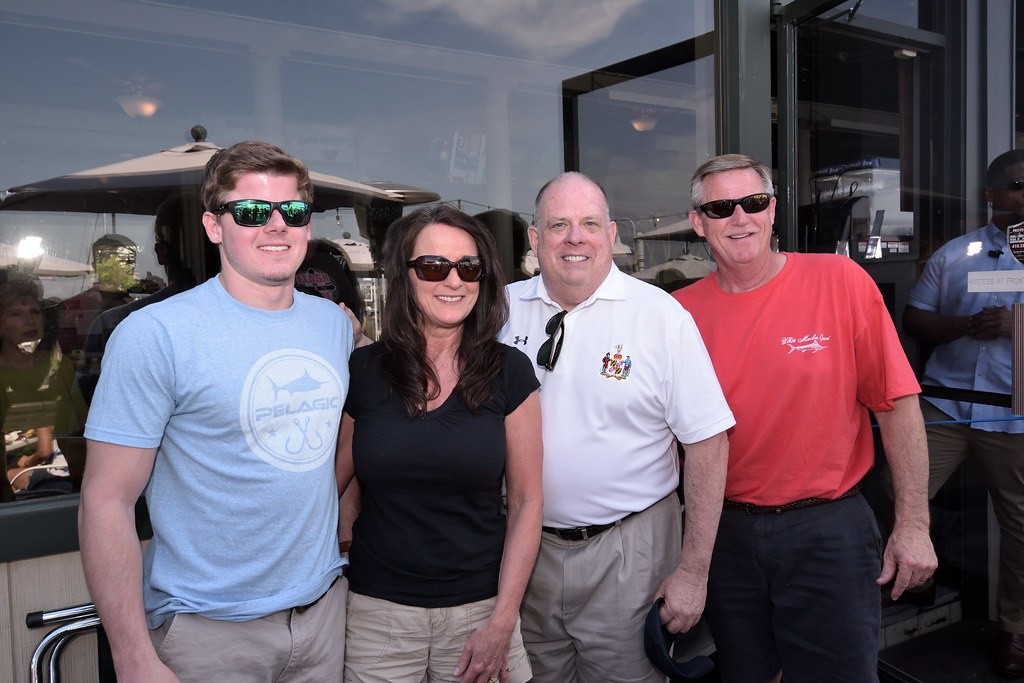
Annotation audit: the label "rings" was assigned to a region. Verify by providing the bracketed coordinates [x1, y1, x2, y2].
[488, 677, 499, 683]
[500, 666, 510, 671]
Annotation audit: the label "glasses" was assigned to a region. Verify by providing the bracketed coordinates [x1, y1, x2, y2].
[696, 192, 775, 221]
[406, 255, 483, 283]
[213, 200, 315, 229]
[538, 310, 570, 371]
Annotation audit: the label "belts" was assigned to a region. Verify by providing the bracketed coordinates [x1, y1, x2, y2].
[724, 483, 860, 517]
[541, 511, 639, 545]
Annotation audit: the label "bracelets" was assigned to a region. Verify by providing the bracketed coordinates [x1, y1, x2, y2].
[339, 541, 354, 552]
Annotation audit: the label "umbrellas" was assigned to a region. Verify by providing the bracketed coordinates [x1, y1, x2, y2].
[0, 126, 404, 283]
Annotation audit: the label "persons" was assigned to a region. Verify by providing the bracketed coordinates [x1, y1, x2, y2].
[336, 200, 545, 683]
[663, 153, 938, 683]
[0, 192, 221, 499]
[496, 171, 737, 682]
[76, 140, 362, 683]
[877, 149, 1024, 677]
